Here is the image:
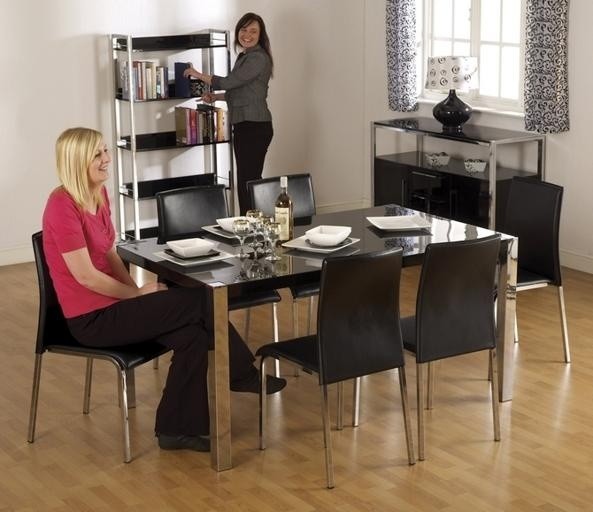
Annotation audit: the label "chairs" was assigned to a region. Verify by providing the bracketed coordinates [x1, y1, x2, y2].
[351, 233, 501, 461]
[153, 183, 282, 379]
[247, 173, 319, 378]
[488, 175, 571, 381]
[26, 231, 186, 463]
[254, 247, 415, 490]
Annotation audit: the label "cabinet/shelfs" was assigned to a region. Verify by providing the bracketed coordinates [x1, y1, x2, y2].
[370, 116, 548, 293]
[110, 27, 239, 242]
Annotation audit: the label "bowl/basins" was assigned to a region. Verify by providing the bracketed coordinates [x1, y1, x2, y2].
[165, 237, 213, 257]
[302, 225, 351, 244]
[215, 216, 255, 233]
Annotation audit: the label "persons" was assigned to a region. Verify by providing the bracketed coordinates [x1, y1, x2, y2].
[40, 127, 287, 454]
[182, 13, 275, 216]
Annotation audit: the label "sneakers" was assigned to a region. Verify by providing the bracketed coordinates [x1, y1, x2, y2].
[231, 368, 286, 393]
[156, 432, 210, 451]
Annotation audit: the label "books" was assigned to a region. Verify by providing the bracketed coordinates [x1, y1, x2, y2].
[173, 103, 227, 145]
[118, 60, 192, 101]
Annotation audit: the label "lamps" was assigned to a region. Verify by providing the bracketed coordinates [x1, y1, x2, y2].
[424, 55, 479, 131]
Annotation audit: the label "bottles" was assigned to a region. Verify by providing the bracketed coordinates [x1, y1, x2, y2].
[272, 176, 294, 245]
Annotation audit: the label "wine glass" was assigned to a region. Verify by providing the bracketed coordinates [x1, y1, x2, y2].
[231, 210, 283, 261]
[231, 256, 272, 282]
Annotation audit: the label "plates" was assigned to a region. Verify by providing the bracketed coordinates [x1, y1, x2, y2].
[282, 247, 360, 260]
[365, 215, 431, 232]
[212, 226, 236, 237]
[163, 248, 219, 260]
[305, 236, 352, 249]
[366, 226, 433, 240]
[200, 223, 261, 239]
[280, 234, 360, 255]
[152, 248, 236, 267]
[156, 259, 234, 275]
